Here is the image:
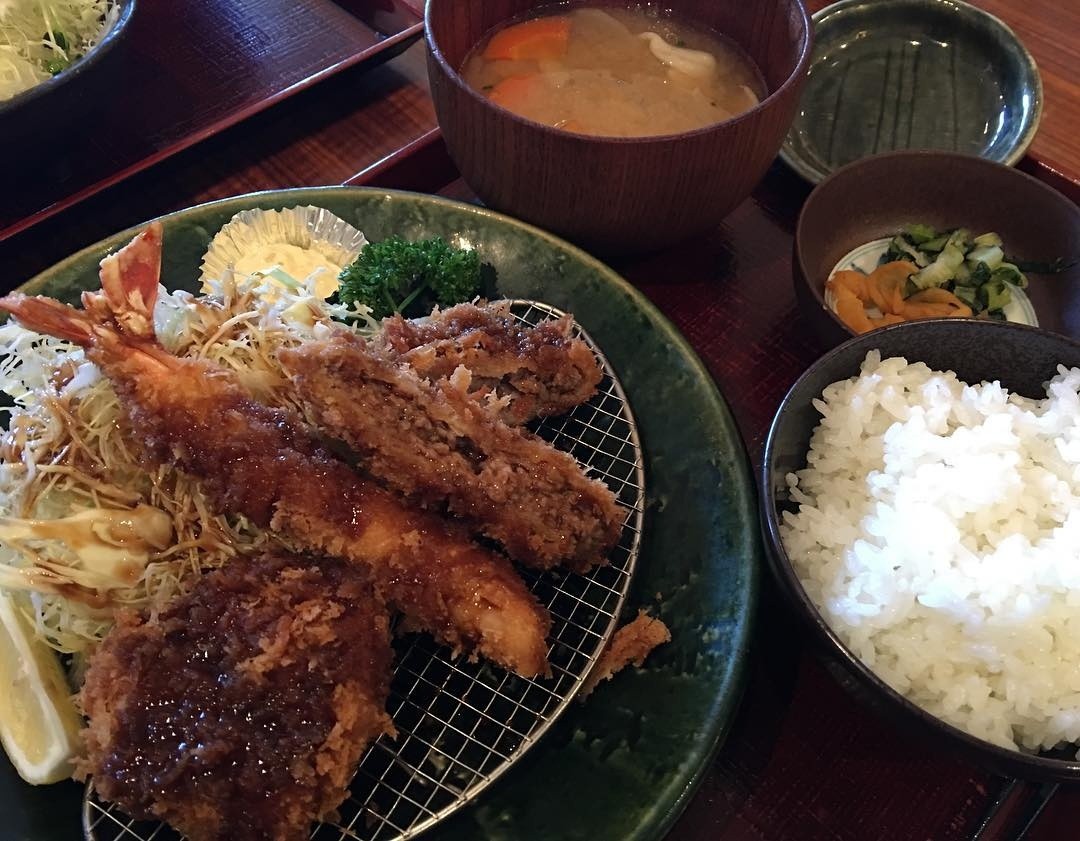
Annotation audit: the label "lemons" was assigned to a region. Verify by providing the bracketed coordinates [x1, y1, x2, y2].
[1, 595, 89, 792]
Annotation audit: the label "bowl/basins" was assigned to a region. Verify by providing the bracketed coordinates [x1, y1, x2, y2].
[419, 1, 814, 250]
[794, 150, 1080, 372]
[750, 312, 1079, 790]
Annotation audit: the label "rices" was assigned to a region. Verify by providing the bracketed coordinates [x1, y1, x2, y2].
[778, 342, 1080, 758]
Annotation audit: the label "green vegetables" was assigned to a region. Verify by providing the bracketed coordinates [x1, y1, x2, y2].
[869, 214, 1028, 321]
[337, 235, 482, 318]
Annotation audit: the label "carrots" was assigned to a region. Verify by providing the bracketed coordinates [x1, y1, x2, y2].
[828, 260, 973, 337]
[486, 12, 571, 57]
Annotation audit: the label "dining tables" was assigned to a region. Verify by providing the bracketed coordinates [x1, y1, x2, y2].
[3, 0, 1080, 838]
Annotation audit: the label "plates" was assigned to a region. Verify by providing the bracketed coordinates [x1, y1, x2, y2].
[0, 0, 138, 115]
[0, 185, 758, 839]
[778, 0, 1045, 195]
